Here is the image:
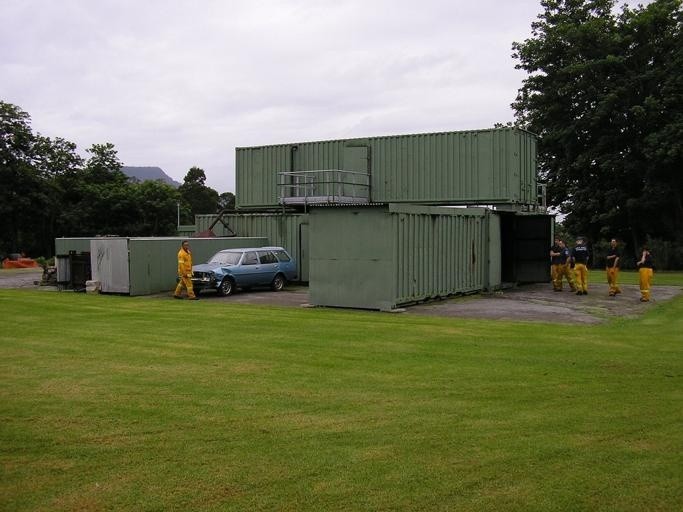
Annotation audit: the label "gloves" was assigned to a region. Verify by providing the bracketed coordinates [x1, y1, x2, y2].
[176, 277, 180, 283]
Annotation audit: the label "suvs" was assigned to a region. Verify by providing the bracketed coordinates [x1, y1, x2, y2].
[176, 245, 298, 296]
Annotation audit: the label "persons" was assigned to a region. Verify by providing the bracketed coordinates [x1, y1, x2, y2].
[636, 246, 655, 302]
[554, 239, 575, 292]
[569, 237, 591, 295]
[604, 237, 621, 296]
[170, 241, 197, 298]
[549, 236, 561, 292]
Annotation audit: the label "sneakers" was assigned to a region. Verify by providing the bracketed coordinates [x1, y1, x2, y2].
[575, 291, 581, 295]
[174, 295, 183, 299]
[569, 288, 576, 292]
[582, 291, 587, 295]
[189, 296, 199, 300]
